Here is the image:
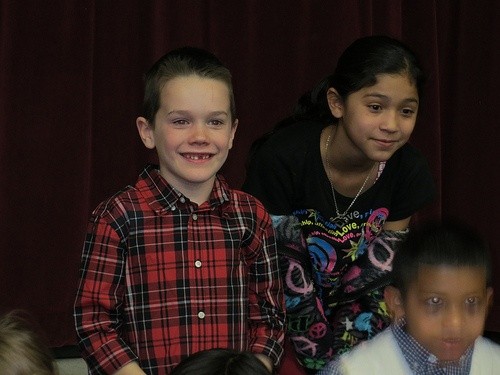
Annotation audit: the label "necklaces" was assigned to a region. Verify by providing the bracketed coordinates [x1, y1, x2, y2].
[324, 126, 376, 222]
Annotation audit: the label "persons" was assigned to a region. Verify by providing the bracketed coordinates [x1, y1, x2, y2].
[244, 34, 432, 375]
[72, 45, 287, 375]
[316, 219, 499, 375]
